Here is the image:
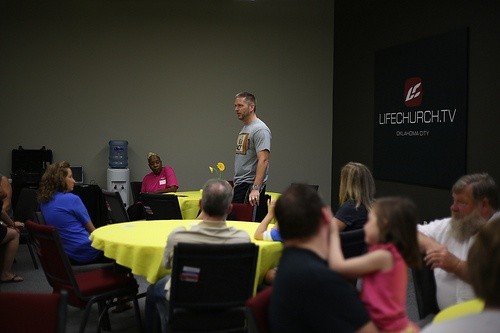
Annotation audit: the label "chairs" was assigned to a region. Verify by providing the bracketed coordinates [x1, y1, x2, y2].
[131, 181, 142, 200]
[244, 286, 272, 333]
[24, 221, 142, 333]
[139, 192, 182, 221]
[232, 203, 257, 222]
[0, 289, 67, 333]
[102, 189, 129, 222]
[10, 190, 41, 269]
[144, 241, 260, 333]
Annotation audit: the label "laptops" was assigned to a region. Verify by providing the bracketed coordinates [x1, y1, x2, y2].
[71, 165, 84, 184]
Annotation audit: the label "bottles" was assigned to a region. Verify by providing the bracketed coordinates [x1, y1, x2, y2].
[108, 140, 129, 169]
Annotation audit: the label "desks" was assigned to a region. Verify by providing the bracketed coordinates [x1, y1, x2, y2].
[88, 220, 284, 333]
[13, 185, 109, 228]
[164, 190, 282, 224]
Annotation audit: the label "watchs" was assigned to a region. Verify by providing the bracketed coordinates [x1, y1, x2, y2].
[252, 184, 262, 190]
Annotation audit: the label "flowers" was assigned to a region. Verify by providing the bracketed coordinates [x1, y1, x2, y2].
[209, 163, 225, 180]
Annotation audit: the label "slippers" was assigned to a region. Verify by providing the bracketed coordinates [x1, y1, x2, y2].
[2, 274, 24, 282]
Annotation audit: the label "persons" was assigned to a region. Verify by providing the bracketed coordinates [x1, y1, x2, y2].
[145, 178, 251, 333]
[421, 217, 500, 333]
[333, 162, 376, 231]
[328, 197, 420, 333]
[254, 199, 281, 242]
[233, 92, 271, 222]
[269, 183, 378, 333]
[416, 173, 496, 310]
[0, 176, 24, 282]
[127, 155, 178, 221]
[38, 160, 133, 313]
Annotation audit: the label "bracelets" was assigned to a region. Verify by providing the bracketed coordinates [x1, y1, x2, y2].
[14, 221, 15, 228]
